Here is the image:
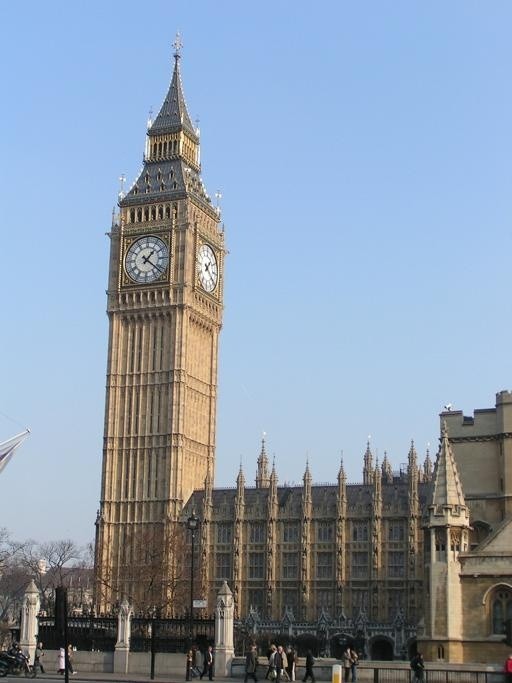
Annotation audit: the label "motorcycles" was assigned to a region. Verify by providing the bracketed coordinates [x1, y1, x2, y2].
[1, 647, 37, 677]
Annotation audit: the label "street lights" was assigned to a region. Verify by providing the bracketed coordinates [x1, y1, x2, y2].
[186, 507, 200, 680]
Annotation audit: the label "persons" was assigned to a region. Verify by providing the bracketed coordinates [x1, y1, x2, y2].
[301, 646, 316, 683]
[31, 641, 47, 673]
[67, 642, 78, 675]
[505, 654, 512, 683]
[350, 649, 359, 683]
[191, 643, 297, 683]
[343, 646, 355, 683]
[8, 642, 28, 671]
[55, 647, 65, 675]
[415, 653, 424, 683]
[409, 652, 426, 683]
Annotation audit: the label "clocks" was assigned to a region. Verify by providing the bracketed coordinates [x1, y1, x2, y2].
[197, 241, 220, 294]
[122, 234, 170, 284]
